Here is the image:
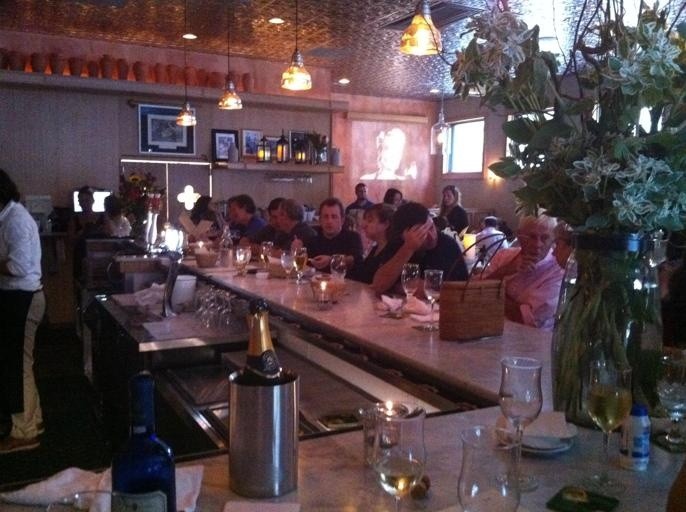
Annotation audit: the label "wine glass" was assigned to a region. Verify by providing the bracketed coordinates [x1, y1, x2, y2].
[359, 406, 385, 511]
[457, 426, 524, 510]
[160, 218, 445, 333]
[374, 402, 426, 510]
[582, 361, 631, 498]
[656, 356, 686, 451]
[494, 357, 545, 490]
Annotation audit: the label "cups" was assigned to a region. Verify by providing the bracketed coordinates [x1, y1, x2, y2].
[46, 485, 127, 511]
[224, 366, 299, 499]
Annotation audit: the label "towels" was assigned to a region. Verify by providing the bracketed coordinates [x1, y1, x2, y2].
[0, 462, 207, 512]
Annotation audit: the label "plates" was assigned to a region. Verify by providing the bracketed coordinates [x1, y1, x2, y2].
[495, 414, 574, 454]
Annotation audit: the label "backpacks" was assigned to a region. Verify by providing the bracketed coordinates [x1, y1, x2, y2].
[437, 232, 510, 343]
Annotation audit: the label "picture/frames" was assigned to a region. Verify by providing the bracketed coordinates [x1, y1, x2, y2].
[240, 127, 263, 158]
[135, 102, 196, 157]
[263, 134, 289, 158]
[210, 127, 241, 163]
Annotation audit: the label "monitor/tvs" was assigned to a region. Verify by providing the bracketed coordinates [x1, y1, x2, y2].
[72, 189, 113, 214]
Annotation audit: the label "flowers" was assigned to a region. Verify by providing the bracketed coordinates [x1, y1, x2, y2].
[116, 168, 167, 239]
[307, 129, 329, 163]
[445, 0, 686, 414]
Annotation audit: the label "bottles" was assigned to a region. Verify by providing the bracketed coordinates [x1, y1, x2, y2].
[333, 150, 342, 166]
[228, 142, 238, 163]
[619, 404, 651, 470]
[0, 48, 255, 93]
[107, 370, 179, 512]
[243, 294, 285, 387]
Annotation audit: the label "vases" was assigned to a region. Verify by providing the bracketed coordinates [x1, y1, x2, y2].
[130, 212, 148, 239]
[0, 46, 257, 96]
[310, 144, 324, 165]
[551, 240, 666, 427]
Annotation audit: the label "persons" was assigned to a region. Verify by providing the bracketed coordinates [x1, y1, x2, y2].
[0, 169, 47, 455]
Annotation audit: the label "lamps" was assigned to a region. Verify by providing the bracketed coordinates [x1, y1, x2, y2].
[273, 128, 289, 163]
[173, 1, 198, 130]
[396, 0, 443, 57]
[279, 1, 312, 89]
[255, 134, 271, 162]
[293, 140, 308, 165]
[430, 50, 452, 157]
[217, 1, 242, 111]
